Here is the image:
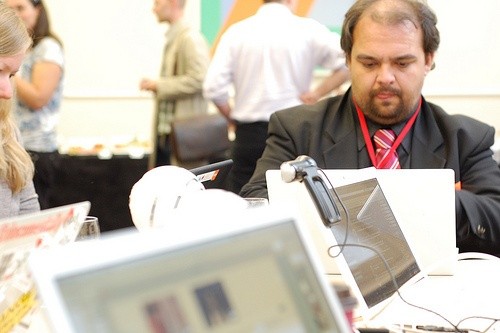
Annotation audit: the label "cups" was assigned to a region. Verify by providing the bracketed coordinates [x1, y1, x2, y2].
[244, 197, 268, 208]
[77, 217, 100, 238]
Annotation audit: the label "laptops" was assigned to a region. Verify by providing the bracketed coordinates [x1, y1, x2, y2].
[30, 203, 355, 333]
[265, 169, 460, 276]
[294, 167, 500, 333]
[0, 201, 91, 333]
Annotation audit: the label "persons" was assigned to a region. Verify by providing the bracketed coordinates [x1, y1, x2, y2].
[239, 0, 500, 255]
[139, 1, 207, 169]
[202, 0, 351, 195]
[7, 0, 66, 209]
[0, 2, 41, 218]
